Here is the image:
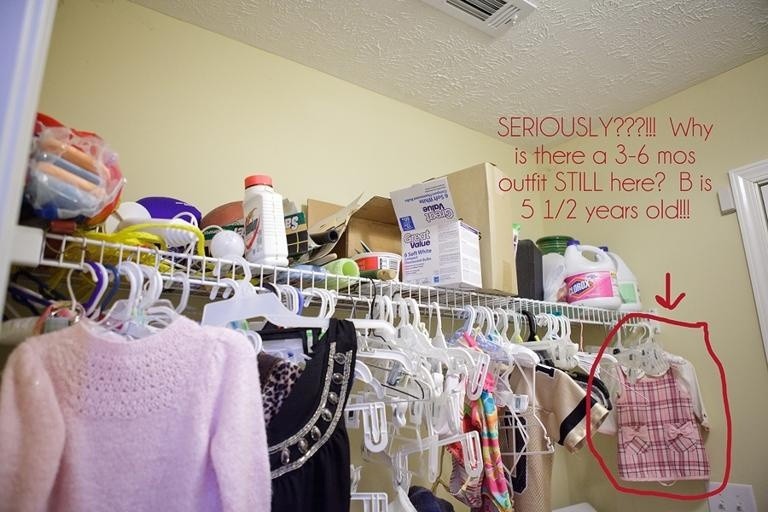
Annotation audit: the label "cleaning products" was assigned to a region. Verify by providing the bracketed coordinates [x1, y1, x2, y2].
[594, 246, 642, 311]
[565, 240, 622, 310]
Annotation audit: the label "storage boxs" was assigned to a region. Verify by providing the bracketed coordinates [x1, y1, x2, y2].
[390, 176, 482, 292]
[307, 195, 402, 259]
[435, 162, 519, 296]
